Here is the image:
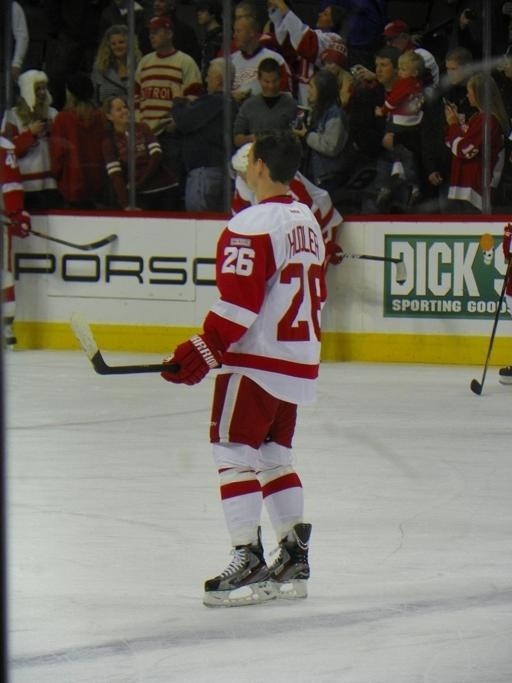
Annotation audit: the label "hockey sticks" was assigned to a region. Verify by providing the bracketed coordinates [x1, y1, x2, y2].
[1, 213, 119, 251]
[69, 312, 182, 376]
[336, 254, 406, 286]
[471, 262, 508, 394]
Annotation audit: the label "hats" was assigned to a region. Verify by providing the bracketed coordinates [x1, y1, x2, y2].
[147, 15, 175, 34]
[328, 242, 346, 266]
[16, 69, 55, 111]
[65, 72, 96, 99]
[320, 37, 351, 68]
[382, 19, 411, 43]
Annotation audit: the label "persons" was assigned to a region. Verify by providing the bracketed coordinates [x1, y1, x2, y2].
[230, 142, 345, 273]
[0, 130, 32, 344]
[499, 222, 512, 385]
[159, 131, 327, 605]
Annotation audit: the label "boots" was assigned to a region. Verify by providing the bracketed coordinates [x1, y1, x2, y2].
[205, 525, 278, 592]
[2, 324, 17, 345]
[263, 523, 314, 582]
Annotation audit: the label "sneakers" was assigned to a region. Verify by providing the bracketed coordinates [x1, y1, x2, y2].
[158, 333, 223, 387]
[377, 188, 395, 208]
[406, 190, 428, 208]
[498, 363, 512, 378]
[7, 210, 32, 237]
